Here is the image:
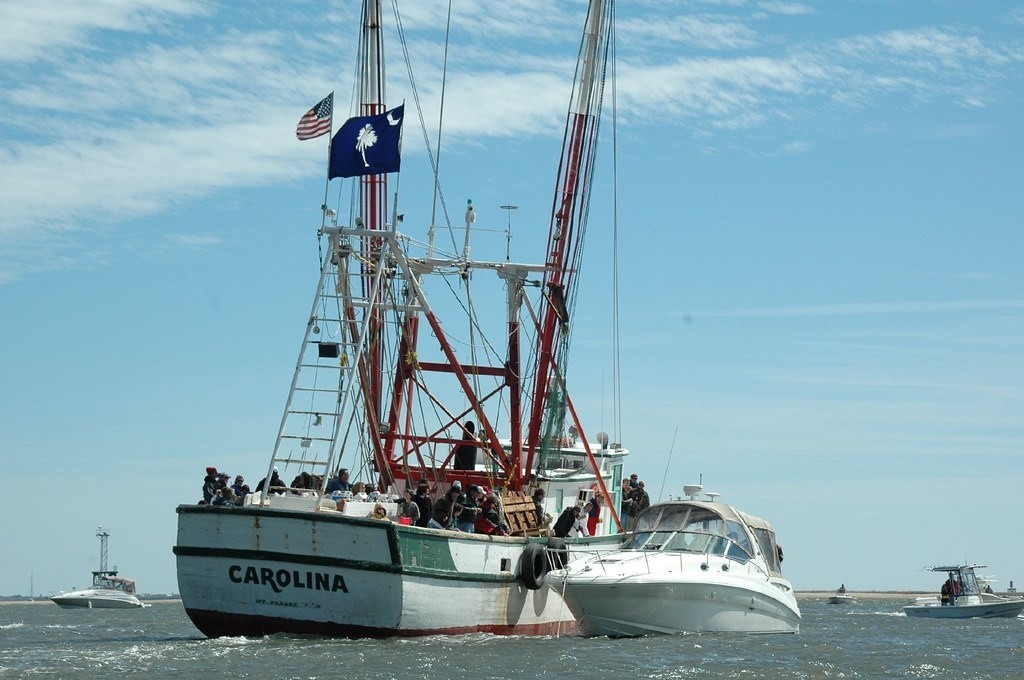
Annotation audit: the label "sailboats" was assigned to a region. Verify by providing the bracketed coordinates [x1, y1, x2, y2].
[172, 0, 630, 641]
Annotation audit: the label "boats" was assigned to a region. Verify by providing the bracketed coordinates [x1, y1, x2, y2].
[541, 473, 802, 639]
[50, 575, 141, 608]
[828, 584, 858, 604]
[902, 560, 1024, 619]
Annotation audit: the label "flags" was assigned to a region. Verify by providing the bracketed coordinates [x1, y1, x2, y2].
[296, 92, 331, 141]
[328, 103, 405, 182]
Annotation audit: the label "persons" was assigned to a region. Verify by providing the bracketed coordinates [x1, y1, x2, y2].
[203, 467, 650, 538]
[940, 581, 969, 607]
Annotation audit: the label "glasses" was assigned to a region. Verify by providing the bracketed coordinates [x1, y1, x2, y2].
[236, 480, 243, 482]
[639, 483, 644, 487]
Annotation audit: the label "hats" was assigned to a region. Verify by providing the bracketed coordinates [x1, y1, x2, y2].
[218, 473, 231, 478]
[470, 484, 480, 494]
[450, 485, 462, 494]
[483, 486, 492, 494]
[477, 486, 487, 495]
[631, 474, 638, 479]
[206, 467, 217, 475]
[273, 466, 278, 473]
